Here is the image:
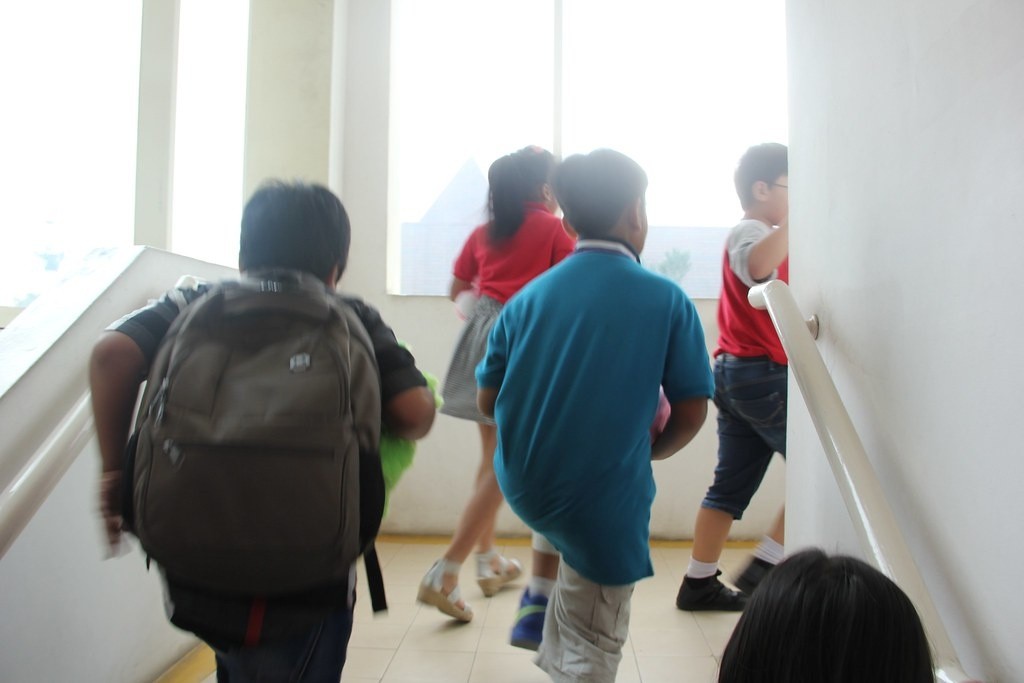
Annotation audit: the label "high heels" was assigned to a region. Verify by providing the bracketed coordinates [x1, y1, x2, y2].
[416, 558, 473, 623]
[476, 551, 524, 597]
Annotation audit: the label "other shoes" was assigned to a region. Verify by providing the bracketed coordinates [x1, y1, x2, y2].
[736, 558, 775, 596]
[675, 569, 751, 612]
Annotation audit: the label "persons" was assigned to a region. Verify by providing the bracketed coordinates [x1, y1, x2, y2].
[88, 180, 435, 683]
[675, 142, 787, 613]
[473, 149, 718, 683]
[418, 144, 576, 623]
[717, 546, 937, 683]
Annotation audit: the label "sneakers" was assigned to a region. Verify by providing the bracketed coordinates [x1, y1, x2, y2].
[509, 590, 548, 650]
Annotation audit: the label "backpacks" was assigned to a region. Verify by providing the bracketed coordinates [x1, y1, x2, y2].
[132, 268, 384, 566]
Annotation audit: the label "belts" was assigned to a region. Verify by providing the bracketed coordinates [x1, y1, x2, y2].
[715, 350, 771, 362]
